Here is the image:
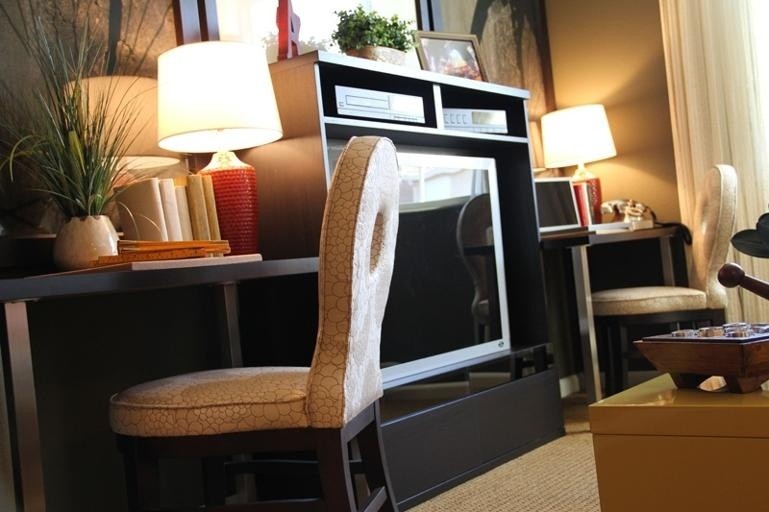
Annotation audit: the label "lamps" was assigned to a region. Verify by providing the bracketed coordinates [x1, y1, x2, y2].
[540, 103, 617, 226]
[155, 39, 285, 258]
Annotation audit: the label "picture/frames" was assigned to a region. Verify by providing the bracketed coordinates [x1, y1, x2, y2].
[411, 30, 490, 83]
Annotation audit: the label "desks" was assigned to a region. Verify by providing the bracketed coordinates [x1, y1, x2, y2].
[540, 224, 680, 406]
[1, 254, 318, 511]
[587, 370, 769, 512]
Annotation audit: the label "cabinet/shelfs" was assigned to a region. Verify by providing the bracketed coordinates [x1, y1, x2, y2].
[235, 51, 566, 511]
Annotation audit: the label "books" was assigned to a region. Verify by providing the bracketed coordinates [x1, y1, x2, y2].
[97, 174, 232, 266]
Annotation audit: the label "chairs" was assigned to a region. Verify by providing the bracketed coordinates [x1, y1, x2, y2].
[590, 163, 741, 399]
[106, 131, 400, 511]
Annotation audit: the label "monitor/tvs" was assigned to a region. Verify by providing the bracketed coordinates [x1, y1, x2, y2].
[534, 176, 583, 233]
[325, 136, 513, 394]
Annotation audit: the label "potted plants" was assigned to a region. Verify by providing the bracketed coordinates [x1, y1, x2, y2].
[1, 1, 176, 270]
[329, 3, 422, 66]
